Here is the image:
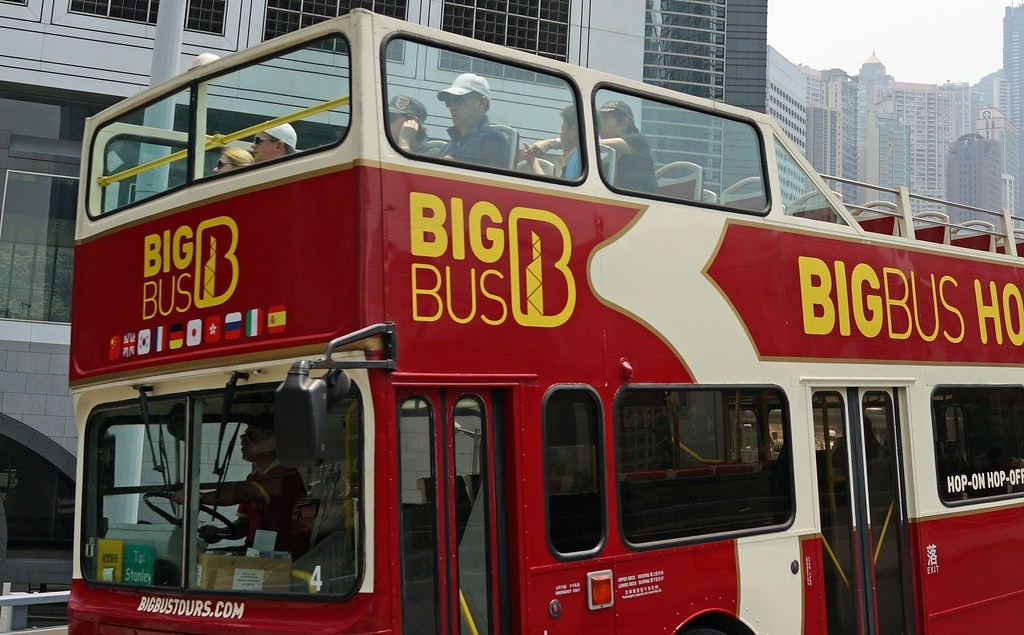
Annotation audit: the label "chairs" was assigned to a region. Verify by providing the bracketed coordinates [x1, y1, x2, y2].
[415, 472, 485, 512]
[430, 124, 1024, 258]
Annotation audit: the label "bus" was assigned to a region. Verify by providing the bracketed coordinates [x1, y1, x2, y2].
[68, 8, 1024, 635]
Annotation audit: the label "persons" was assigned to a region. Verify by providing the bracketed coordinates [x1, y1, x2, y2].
[213, 122, 297, 173]
[172, 411, 313, 563]
[388, 72, 659, 194]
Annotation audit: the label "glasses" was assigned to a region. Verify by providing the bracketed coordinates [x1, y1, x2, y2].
[254, 136, 277, 144]
[217, 160, 236, 169]
[240, 433, 272, 444]
[386, 111, 404, 124]
[444, 94, 481, 107]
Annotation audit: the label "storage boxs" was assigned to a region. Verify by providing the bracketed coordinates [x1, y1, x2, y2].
[195, 553, 292, 593]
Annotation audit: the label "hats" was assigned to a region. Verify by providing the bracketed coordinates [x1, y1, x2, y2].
[597, 99, 639, 123]
[437, 73, 491, 101]
[387, 94, 428, 124]
[265, 120, 298, 155]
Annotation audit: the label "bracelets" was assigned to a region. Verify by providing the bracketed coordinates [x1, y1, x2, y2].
[200, 492, 205, 505]
